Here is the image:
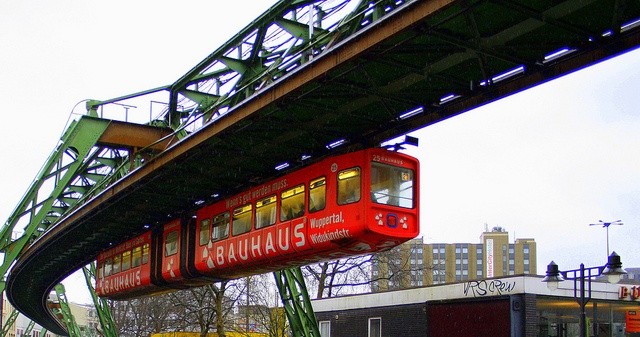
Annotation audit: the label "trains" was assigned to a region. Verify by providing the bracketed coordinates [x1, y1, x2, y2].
[94, 135, 420, 301]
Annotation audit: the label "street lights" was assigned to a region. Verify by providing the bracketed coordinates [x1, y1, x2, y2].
[541, 251, 628, 337]
